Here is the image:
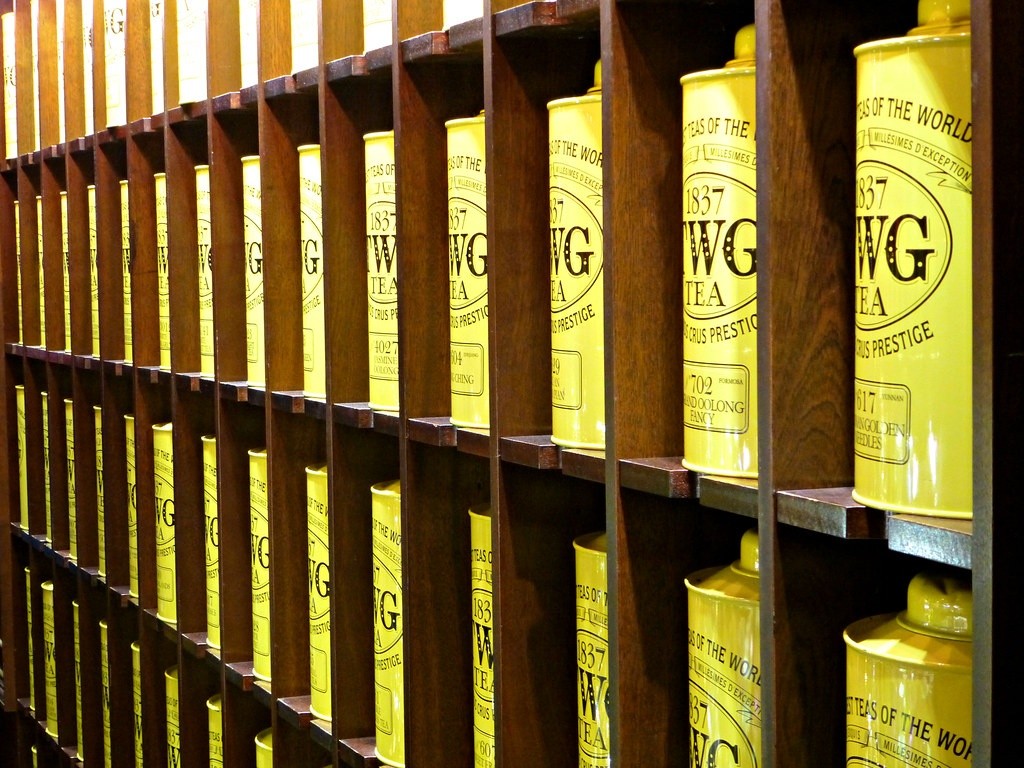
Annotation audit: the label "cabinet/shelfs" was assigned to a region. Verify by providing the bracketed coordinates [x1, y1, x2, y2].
[2, 0, 1023, 768]
